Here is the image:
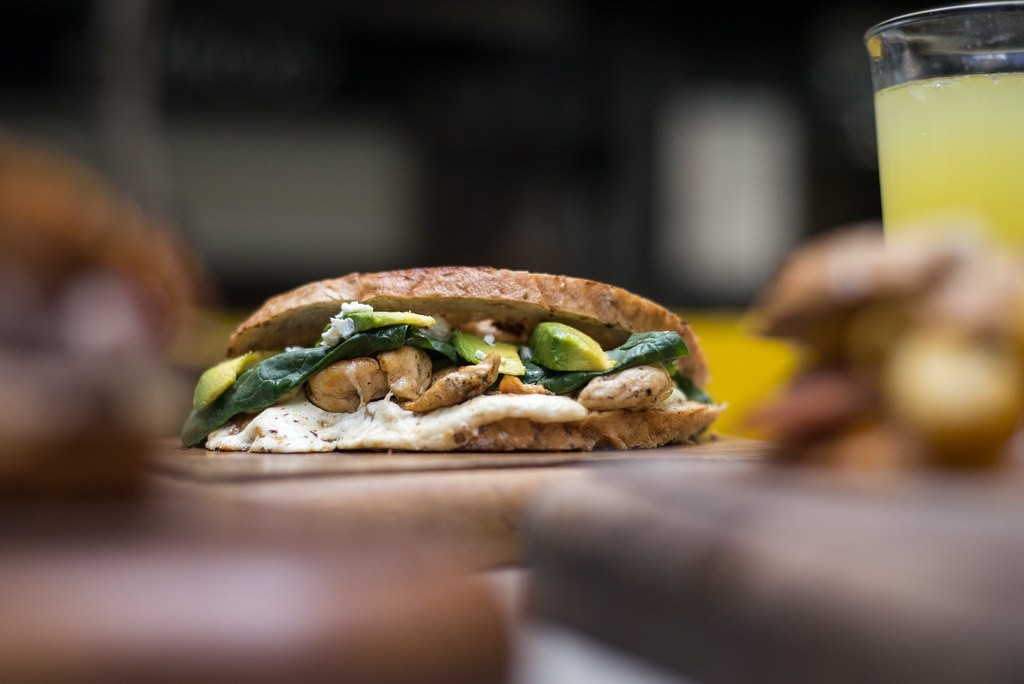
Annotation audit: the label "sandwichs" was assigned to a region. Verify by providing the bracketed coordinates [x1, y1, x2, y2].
[197, 269, 721, 451]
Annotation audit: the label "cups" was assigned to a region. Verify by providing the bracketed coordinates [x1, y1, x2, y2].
[861, 0, 1024, 256]
